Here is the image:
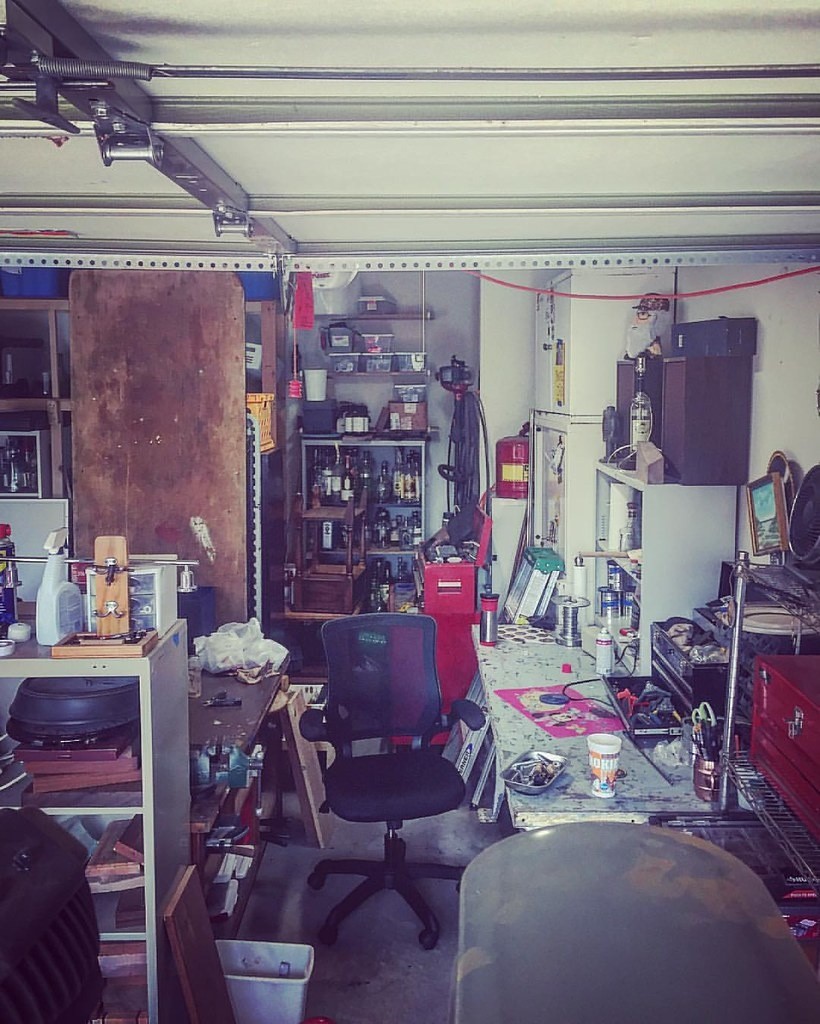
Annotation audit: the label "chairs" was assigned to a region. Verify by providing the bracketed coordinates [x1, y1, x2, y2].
[450, 820, 819, 1024]
[297, 613, 466, 949]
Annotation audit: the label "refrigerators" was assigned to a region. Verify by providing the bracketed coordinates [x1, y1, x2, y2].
[523, 267, 676, 629]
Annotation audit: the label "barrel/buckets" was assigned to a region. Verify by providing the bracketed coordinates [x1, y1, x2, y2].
[692, 755, 723, 803]
[304, 369, 327, 402]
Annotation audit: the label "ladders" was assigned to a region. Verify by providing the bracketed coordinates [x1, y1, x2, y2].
[443, 548, 565, 810]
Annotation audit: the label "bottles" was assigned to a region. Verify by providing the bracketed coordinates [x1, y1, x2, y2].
[0, 445, 38, 493]
[619, 503, 641, 553]
[595, 627, 617, 675]
[284, 447, 422, 612]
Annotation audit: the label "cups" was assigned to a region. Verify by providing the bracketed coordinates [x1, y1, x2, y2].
[586, 733, 622, 799]
[479, 592, 500, 646]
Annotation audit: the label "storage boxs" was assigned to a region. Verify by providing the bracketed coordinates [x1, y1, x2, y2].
[326, 326, 355, 354]
[302, 403, 335, 434]
[357, 295, 396, 315]
[388, 401, 427, 431]
[328, 352, 361, 375]
[360, 334, 396, 353]
[304, 369, 328, 401]
[363, 353, 393, 373]
[395, 384, 426, 403]
[393, 351, 426, 371]
[216, 939, 314, 1024]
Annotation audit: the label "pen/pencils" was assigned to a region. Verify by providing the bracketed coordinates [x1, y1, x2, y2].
[690, 719, 720, 759]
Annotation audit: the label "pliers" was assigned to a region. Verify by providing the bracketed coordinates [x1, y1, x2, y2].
[201, 689, 242, 706]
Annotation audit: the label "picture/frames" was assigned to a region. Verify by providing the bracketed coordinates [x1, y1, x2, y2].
[744, 471, 790, 557]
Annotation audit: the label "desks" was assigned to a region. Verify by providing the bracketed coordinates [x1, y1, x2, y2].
[473, 621, 751, 831]
[188, 651, 292, 836]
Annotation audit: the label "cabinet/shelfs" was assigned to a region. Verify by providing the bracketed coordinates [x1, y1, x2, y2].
[0, 619, 190, 1024]
[0, 300, 72, 498]
[591, 461, 736, 677]
[615, 361, 660, 464]
[301, 435, 425, 568]
[660, 353, 752, 485]
[748, 650, 820, 843]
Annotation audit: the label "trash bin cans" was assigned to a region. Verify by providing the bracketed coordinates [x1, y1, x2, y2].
[216, 936, 314, 1024]
